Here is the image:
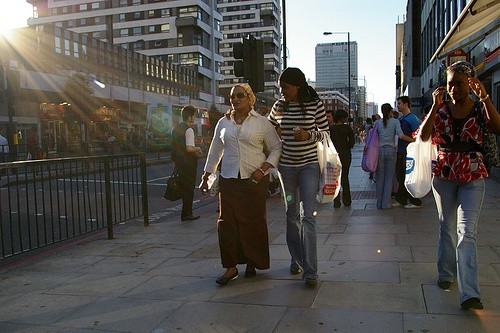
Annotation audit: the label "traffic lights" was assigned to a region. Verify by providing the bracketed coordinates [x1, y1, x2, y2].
[233, 32, 265, 93]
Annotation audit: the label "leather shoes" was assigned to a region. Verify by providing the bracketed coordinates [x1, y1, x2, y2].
[181, 215, 201, 221]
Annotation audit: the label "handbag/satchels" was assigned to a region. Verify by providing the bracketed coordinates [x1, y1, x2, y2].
[477, 104, 500, 168]
[404, 132, 436, 199]
[315, 133, 342, 203]
[362, 120, 379, 172]
[165, 166, 182, 201]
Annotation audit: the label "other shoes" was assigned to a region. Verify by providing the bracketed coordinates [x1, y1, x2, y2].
[306, 278, 317, 286]
[461, 297, 482, 309]
[290, 264, 301, 273]
[404, 204, 424, 208]
[245, 268, 255, 277]
[438, 279, 449, 289]
[392, 202, 403, 207]
[216, 268, 238, 285]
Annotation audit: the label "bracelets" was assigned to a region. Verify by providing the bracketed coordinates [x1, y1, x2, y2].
[480, 94, 489, 102]
[203, 176, 209, 180]
[257, 168, 264, 175]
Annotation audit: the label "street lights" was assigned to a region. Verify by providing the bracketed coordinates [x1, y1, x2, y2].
[323, 31, 351, 128]
[352, 75, 365, 126]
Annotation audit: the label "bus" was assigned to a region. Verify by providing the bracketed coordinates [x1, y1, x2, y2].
[145, 103, 212, 157]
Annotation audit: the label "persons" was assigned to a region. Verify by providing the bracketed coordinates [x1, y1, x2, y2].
[226, 67, 330, 287]
[200, 83, 282, 285]
[254, 106, 281, 199]
[0, 134, 9, 176]
[27, 126, 42, 172]
[365, 96, 433, 210]
[171, 105, 200, 221]
[326, 109, 355, 208]
[474, 101, 500, 176]
[421, 62, 500, 309]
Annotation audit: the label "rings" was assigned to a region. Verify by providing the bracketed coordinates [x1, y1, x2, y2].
[255, 181, 258, 184]
[252, 179, 255, 182]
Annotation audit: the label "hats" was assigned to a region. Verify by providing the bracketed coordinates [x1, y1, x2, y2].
[381, 104, 393, 110]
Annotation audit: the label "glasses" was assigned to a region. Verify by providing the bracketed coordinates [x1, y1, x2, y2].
[228, 92, 248, 98]
